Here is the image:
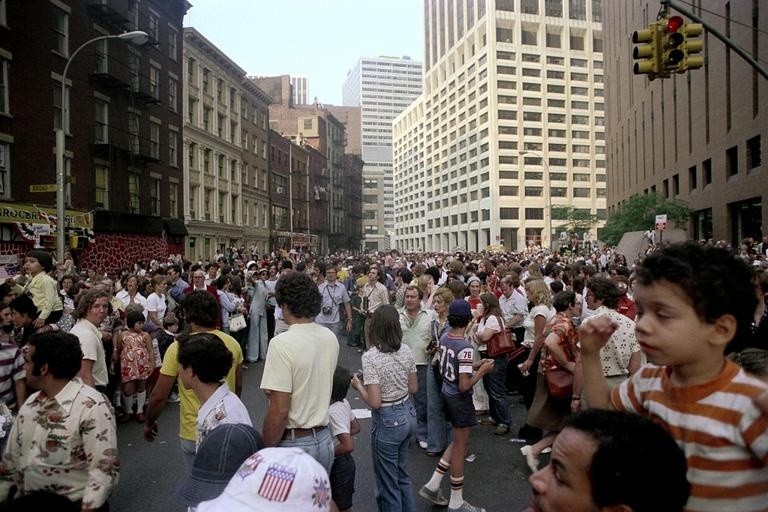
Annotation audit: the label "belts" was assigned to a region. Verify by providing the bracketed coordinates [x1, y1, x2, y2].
[380, 393, 410, 407]
[282, 425, 328, 437]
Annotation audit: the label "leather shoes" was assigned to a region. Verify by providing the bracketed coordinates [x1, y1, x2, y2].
[135, 412, 145, 423]
[419, 442, 428, 448]
[427, 452, 438, 457]
[117, 412, 134, 423]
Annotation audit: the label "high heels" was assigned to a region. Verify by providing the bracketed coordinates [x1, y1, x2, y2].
[520, 445, 540, 473]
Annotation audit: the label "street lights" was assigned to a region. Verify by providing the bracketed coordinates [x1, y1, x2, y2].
[518, 151, 552, 252]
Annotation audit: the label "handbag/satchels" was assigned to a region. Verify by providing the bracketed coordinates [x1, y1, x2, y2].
[363, 296, 369, 309]
[229, 312, 247, 332]
[545, 367, 573, 400]
[339, 302, 352, 321]
[485, 329, 514, 357]
[737, 347, 767, 375]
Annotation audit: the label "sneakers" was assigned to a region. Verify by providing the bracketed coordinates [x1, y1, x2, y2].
[417, 485, 449, 506]
[494, 423, 509, 435]
[447, 500, 485, 512]
[518, 426, 543, 441]
[167, 392, 180, 403]
[479, 417, 497, 425]
[115, 405, 124, 417]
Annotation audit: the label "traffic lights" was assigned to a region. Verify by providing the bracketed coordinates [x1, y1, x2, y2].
[633, 28, 656, 80]
[658, 15, 684, 73]
[680, 21, 704, 70]
[56, 31, 149, 280]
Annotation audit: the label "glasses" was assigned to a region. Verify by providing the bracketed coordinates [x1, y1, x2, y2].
[166, 272, 175, 276]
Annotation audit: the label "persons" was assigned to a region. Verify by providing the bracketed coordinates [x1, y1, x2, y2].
[261, 272, 339, 473]
[0, 331, 120, 511]
[0, 224, 768, 458]
[418, 293, 497, 512]
[527, 409, 691, 512]
[577, 242, 767, 510]
[353, 303, 418, 512]
[141, 293, 245, 477]
[329, 366, 362, 512]
[521, 290, 580, 474]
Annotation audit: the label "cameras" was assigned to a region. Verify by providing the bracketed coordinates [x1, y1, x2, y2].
[322, 306, 333, 314]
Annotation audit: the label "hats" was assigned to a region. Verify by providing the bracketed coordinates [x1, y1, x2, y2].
[466, 276, 481, 287]
[470, 259, 478, 265]
[175, 423, 266, 504]
[246, 260, 257, 269]
[449, 299, 471, 316]
[196, 446, 333, 512]
[259, 267, 268, 275]
[289, 249, 299, 255]
